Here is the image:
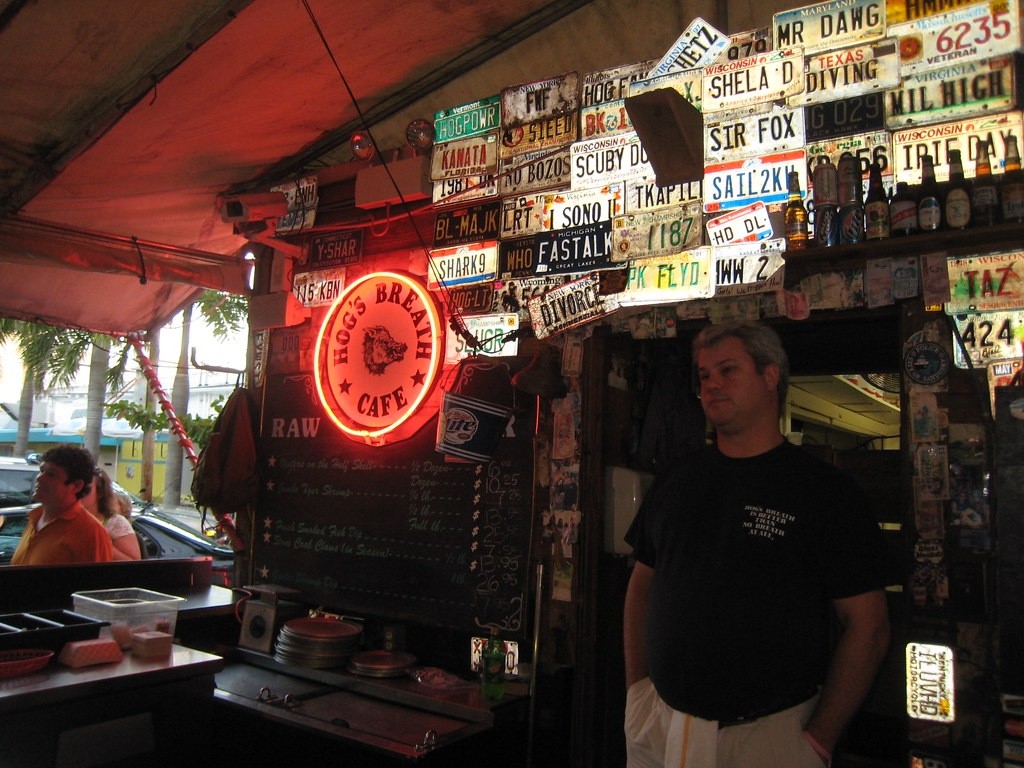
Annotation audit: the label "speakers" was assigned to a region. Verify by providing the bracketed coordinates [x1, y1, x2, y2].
[623, 87, 703, 188]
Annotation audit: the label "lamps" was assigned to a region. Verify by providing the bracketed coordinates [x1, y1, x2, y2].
[350, 119, 435, 210]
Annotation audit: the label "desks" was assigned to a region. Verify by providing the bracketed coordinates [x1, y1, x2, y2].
[0, 642, 224, 768]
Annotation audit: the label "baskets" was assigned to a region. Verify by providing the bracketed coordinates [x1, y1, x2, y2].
[0, 649, 55, 677]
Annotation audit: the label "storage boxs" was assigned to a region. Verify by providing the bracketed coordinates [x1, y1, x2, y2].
[132, 631, 174, 659]
[73, 585, 188, 653]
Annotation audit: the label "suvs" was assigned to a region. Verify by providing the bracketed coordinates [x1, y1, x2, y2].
[0, 452, 160, 513]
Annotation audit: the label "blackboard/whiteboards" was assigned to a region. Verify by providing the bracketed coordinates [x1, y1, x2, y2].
[248, 359, 536, 639]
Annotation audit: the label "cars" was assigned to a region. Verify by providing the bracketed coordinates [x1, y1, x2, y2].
[0, 498, 235, 589]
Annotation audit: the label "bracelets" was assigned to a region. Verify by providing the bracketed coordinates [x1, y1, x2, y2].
[802, 729, 832, 759]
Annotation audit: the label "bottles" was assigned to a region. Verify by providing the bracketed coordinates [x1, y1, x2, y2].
[971, 142, 1000, 228]
[890, 182, 917, 235]
[942, 150, 971, 229]
[916, 155, 942, 231]
[482, 628, 504, 699]
[1000, 136, 1024, 222]
[865, 162, 890, 241]
[783, 172, 807, 251]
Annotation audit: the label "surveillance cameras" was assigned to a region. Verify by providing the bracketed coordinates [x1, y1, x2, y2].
[217, 190, 290, 223]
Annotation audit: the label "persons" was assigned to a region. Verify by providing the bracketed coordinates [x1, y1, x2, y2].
[83, 467, 141, 561]
[113, 494, 149, 559]
[622, 322, 893, 768]
[11, 443, 112, 566]
[542, 457, 579, 544]
